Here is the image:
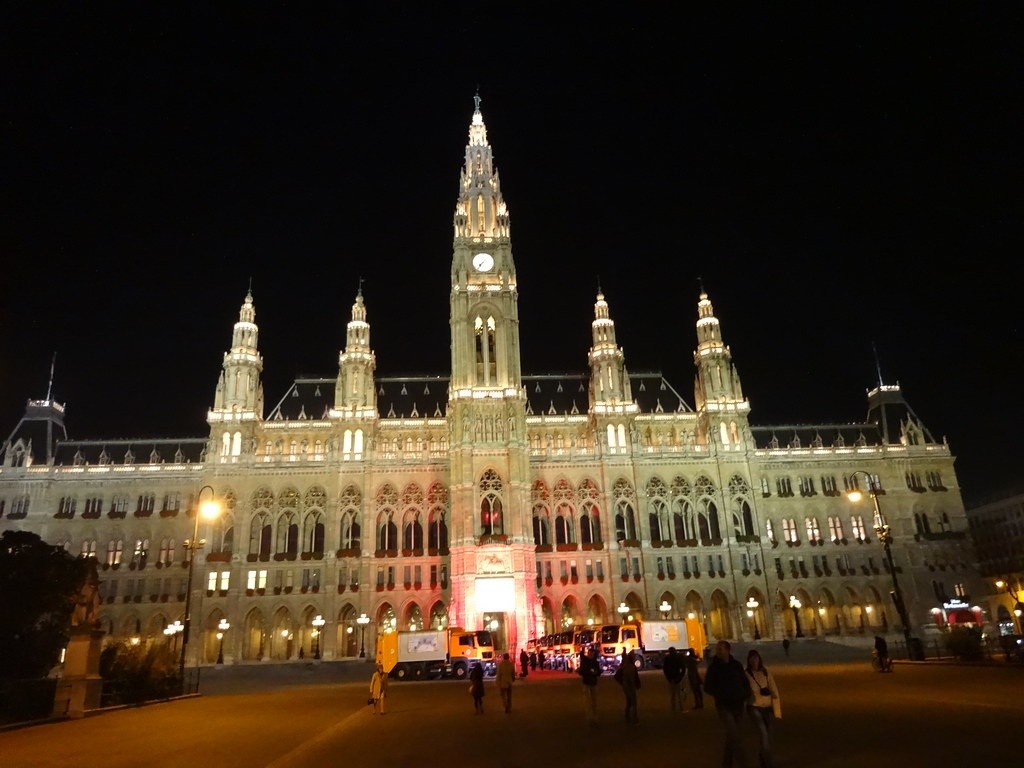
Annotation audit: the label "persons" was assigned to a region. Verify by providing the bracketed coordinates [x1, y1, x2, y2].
[539, 650, 545, 670]
[520, 648, 529, 677]
[370, 665, 388, 715]
[470, 662, 484, 716]
[683, 649, 704, 710]
[783, 636, 789, 657]
[985, 634, 992, 657]
[663, 647, 689, 714]
[875, 635, 889, 672]
[703, 647, 709, 662]
[529, 652, 536, 670]
[578, 649, 604, 729]
[703, 640, 753, 768]
[743, 648, 783, 768]
[619, 646, 641, 726]
[299, 647, 304, 659]
[496, 653, 515, 714]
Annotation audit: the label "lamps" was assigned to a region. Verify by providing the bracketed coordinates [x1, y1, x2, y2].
[627, 613, 633, 621]
[865, 603, 871, 613]
[688, 609, 694, 619]
[819, 606, 825, 615]
[216, 629, 223, 639]
[437, 617, 443, 631]
[281, 609, 289, 636]
[566, 609, 573, 624]
[588, 599, 594, 625]
[346, 623, 353, 633]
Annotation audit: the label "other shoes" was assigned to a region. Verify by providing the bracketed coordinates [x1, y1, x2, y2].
[504, 706, 511, 714]
[624, 716, 640, 727]
[692, 704, 704, 710]
[380, 712, 383, 715]
[372, 712, 376, 715]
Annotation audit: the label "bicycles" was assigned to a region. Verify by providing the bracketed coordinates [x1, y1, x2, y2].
[872, 648, 894, 672]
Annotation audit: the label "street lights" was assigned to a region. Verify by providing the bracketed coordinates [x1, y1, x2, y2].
[618, 603, 631, 625]
[788, 595, 805, 638]
[163, 620, 186, 653]
[312, 615, 326, 659]
[178, 485, 220, 694]
[848, 470, 915, 661]
[357, 613, 370, 658]
[746, 597, 761, 640]
[659, 600, 672, 621]
[216, 618, 231, 664]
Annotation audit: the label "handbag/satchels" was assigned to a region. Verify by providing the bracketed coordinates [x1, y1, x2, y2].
[368, 692, 374, 705]
[613, 664, 624, 685]
[760, 687, 771, 696]
[468, 684, 475, 694]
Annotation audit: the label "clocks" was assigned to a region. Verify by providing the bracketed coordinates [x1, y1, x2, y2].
[472, 253, 494, 272]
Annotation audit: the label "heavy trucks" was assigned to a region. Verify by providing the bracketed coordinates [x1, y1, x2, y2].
[375, 626, 498, 682]
[525, 620, 689, 674]
